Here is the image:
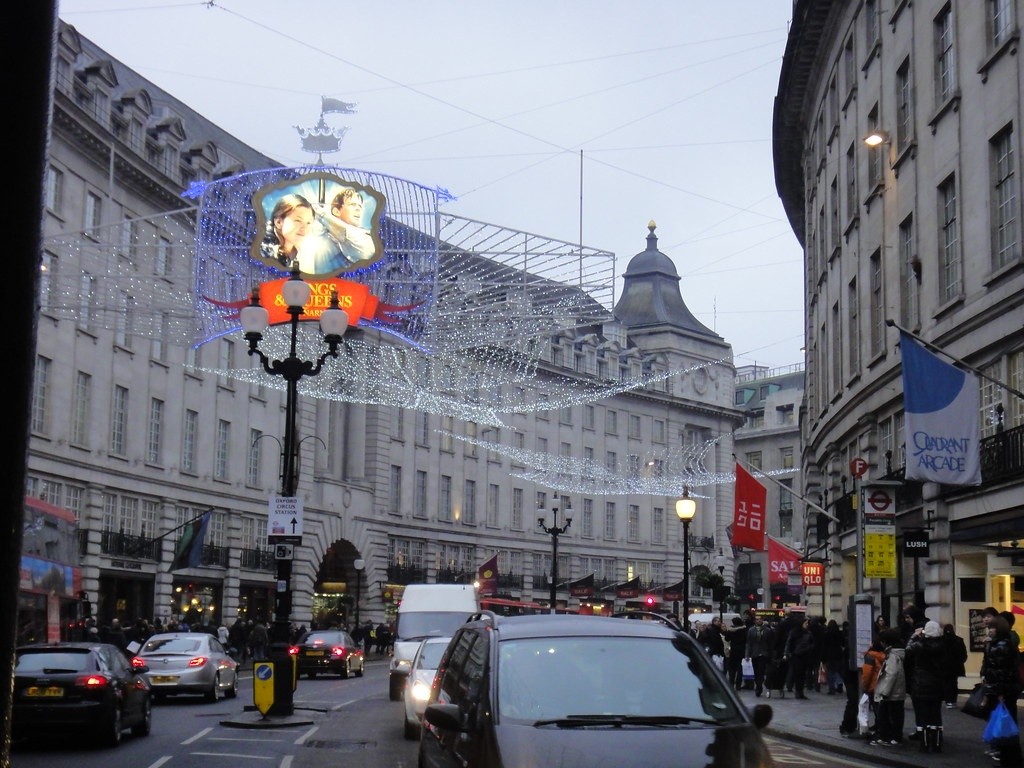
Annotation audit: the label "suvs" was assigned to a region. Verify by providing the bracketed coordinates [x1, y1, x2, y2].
[416, 608, 776, 768]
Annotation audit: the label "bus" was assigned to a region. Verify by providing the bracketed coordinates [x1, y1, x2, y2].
[16, 495, 92, 646]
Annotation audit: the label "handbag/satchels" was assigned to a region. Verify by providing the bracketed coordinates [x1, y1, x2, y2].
[858, 693, 875, 735]
[983, 703, 1001, 743]
[993, 703, 1019, 738]
[126, 641, 141, 655]
[713, 655, 724, 672]
[742, 658, 755, 679]
[961, 683, 997, 721]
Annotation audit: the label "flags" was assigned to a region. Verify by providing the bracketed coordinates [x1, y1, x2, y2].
[733, 462, 767, 552]
[570, 574, 595, 597]
[617, 576, 640, 598]
[479, 556, 498, 593]
[663, 582, 684, 601]
[726, 524, 744, 558]
[167, 512, 211, 572]
[901, 334, 983, 486]
[768, 537, 804, 582]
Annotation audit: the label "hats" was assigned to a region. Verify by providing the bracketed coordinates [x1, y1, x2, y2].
[922, 621, 942, 637]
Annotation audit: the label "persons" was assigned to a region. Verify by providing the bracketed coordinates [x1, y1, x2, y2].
[230, 618, 314, 662]
[83, 615, 229, 648]
[861, 607, 968, 752]
[980, 607, 1021, 760]
[261, 189, 375, 274]
[336, 620, 398, 656]
[667, 608, 853, 699]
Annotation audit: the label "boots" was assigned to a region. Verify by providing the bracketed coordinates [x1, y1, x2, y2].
[917, 725, 944, 754]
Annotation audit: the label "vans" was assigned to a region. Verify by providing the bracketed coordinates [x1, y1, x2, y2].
[389, 583, 481, 701]
[688, 613, 741, 632]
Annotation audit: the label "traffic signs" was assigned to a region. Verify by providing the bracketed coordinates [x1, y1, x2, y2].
[268, 496, 304, 547]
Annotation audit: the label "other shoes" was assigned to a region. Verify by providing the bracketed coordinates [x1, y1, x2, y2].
[869, 739, 901, 746]
[947, 702, 957, 709]
[909, 732, 917, 739]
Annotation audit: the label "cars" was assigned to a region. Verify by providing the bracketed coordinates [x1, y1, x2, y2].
[130, 632, 242, 704]
[13, 642, 153, 751]
[291, 629, 365, 679]
[397, 637, 453, 741]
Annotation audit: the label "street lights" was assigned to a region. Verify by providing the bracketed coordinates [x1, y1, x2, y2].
[238, 259, 349, 716]
[715, 548, 726, 623]
[354, 555, 366, 629]
[537, 491, 575, 615]
[675, 484, 696, 634]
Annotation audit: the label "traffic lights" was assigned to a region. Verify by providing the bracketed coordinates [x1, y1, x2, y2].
[648, 598, 653, 612]
[775, 593, 783, 609]
[748, 593, 757, 610]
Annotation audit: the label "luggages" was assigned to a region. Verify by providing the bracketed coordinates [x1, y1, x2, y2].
[764, 658, 784, 699]
